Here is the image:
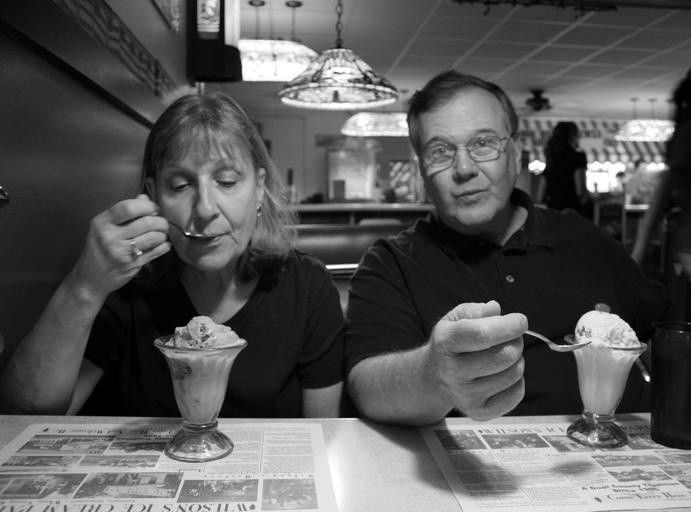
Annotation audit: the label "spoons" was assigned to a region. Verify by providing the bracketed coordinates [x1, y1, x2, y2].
[524, 329, 593, 353]
[168, 221, 218, 241]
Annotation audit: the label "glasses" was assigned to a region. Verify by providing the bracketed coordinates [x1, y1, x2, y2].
[421, 132, 515, 167]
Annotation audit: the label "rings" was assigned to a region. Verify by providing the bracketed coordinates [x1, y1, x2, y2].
[129, 240, 143, 257]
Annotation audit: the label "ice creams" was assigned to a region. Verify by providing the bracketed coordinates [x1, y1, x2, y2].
[574, 304, 643, 414]
[163, 315, 245, 424]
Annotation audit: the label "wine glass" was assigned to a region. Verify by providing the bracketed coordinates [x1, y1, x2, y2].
[563, 334, 648, 449]
[154, 334, 247, 463]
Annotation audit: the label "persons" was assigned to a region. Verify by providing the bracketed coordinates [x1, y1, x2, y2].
[1, 90, 346, 418]
[631, 66, 690, 278]
[540, 120, 618, 236]
[344, 65, 654, 429]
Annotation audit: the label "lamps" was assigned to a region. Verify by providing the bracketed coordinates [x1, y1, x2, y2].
[613, 96, 676, 142]
[230, 0, 319, 84]
[278, 0, 403, 112]
